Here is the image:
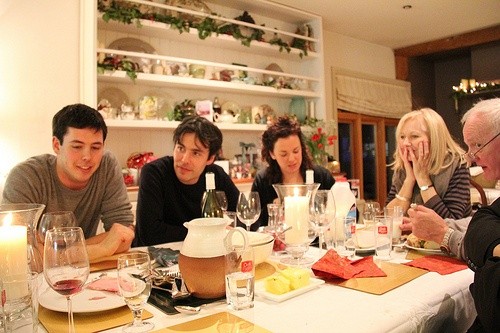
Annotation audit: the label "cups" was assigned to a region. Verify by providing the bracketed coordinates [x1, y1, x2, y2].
[224, 246, 255, 311]
[201, 191, 227, 218]
[0, 271, 39, 333]
[332, 217, 355, 258]
[267, 204, 280, 228]
[361, 203, 403, 258]
[37, 211, 78, 251]
[0, 203, 45, 326]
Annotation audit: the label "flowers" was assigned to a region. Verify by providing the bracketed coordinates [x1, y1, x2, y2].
[306, 128, 337, 163]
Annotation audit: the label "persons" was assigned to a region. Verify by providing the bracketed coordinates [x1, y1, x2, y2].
[0, 103, 135, 272]
[398, 97, 500, 333]
[383, 107, 473, 221]
[250, 114, 360, 228]
[131, 115, 258, 250]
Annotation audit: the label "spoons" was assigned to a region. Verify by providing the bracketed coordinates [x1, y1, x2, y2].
[173, 301, 226, 315]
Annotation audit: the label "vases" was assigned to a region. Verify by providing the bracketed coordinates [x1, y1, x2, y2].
[312, 151, 327, 167]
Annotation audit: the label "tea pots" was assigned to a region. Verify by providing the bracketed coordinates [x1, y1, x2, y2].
[214, 110, 240, 123]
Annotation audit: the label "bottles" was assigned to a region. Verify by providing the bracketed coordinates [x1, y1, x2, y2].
[202, 172, 223, 218]
[329, 176, 356, 242]
[213, 97, 221, 121]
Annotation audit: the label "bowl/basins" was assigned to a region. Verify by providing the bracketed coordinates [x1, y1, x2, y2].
[263, 226, 285, 251]
[233, 231, 274, 268]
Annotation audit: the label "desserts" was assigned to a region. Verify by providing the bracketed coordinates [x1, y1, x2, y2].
[265, 267, 311, 295]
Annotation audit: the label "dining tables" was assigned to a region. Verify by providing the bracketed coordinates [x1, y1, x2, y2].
[0, 214, 477, 333]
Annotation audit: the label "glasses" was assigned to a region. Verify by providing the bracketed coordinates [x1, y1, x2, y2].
[462, 134, 500, 161]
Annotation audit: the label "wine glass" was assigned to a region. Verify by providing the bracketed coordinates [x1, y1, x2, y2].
[271, 184, 322, 265]
[306, 189, 336, 258]
[237, 191, 262, 233]
[43, 227, 90, 333]
[117, 252, 155, 333]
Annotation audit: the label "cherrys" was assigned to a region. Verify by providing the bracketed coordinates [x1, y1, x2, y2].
[273, 233, 286, 251]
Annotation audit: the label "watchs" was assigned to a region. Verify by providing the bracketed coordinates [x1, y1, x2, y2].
[418, 184, 434, 191]
[440, 229, 455, 256]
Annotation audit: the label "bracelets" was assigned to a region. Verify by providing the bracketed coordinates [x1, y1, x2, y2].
[394, 194, 411, 202]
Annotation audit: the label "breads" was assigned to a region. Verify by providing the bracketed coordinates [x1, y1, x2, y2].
[406, 233, 442, 250]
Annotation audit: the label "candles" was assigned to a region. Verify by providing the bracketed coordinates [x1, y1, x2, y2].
[0, 210, 28, 299]
[284, 187, 310, 251]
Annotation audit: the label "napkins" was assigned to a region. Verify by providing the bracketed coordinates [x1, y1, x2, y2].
[311, 248, 388, 281]
[405, 253, 469, 275]
[270, 234, 286, 252]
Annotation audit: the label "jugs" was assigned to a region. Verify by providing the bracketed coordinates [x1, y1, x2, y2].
[178, 217, 249, 298]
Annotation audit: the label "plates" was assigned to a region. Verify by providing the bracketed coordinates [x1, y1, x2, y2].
[254, 277, 325, 303]
[38, 272, 146, 312]
[403, 241, 442, 254]
[356, 234, 390, 255]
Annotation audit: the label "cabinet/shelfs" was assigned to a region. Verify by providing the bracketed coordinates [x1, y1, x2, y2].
[80, 0, 328, 206]
